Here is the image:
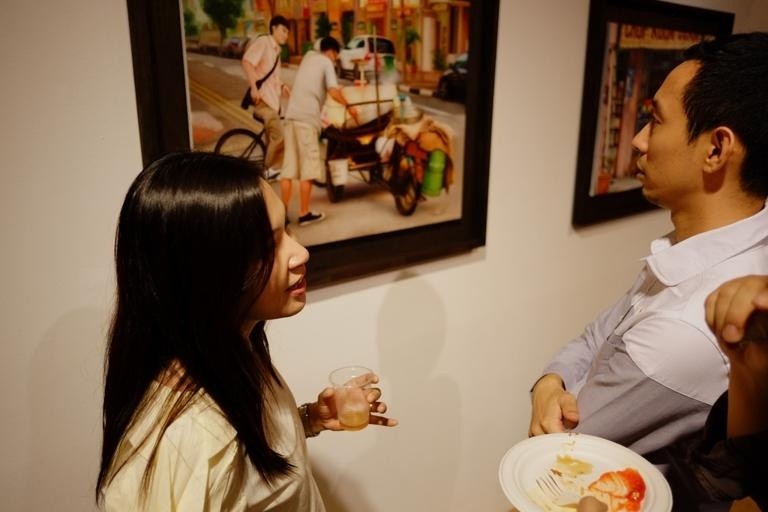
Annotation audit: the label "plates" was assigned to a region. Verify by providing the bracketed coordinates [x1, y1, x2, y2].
[497, 431, 672, 512]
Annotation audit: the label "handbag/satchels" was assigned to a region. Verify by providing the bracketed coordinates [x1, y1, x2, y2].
[241, 79, 261, 109]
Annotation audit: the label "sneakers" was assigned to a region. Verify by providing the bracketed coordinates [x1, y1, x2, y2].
[264, 168, 280, 179]
[298, 212, 325, 226]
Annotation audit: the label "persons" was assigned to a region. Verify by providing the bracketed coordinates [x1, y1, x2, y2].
[666, 274, 768, 512]
[92, 147, 400, 511]
[530, 30, 768, 455]
[242, 15, 292, 179]
[280, 36, 363, 227]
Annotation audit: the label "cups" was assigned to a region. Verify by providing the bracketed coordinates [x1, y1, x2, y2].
[328, 366, 376, 431]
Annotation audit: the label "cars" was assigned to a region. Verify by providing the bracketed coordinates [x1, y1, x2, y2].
[334, 33, 397, 82]
[436, 51, 468, 101]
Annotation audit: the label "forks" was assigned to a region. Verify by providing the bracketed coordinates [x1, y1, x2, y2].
[535, 471, 581, 507]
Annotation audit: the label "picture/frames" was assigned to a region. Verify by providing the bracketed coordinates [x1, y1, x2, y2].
[127, 0, 500, 289]
[572, 0, 735, 226]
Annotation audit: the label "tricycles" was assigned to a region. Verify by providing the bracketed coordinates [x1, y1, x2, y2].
[321, 98, 422, 215]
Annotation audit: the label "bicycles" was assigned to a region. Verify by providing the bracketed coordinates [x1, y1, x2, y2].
[213, 112, 328, 188]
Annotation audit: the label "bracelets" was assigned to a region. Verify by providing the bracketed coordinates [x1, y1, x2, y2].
[299, 402, 320, 445]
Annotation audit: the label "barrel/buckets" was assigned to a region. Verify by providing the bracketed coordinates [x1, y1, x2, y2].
[328, 158, 349, 185]
